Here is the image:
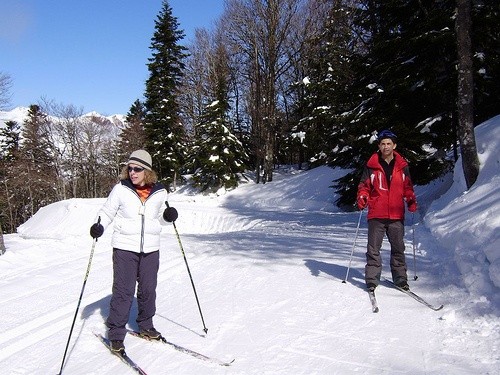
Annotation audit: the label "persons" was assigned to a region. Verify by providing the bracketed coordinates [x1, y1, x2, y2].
[90, 150, 179, 352]
[357, 131, 418, 290]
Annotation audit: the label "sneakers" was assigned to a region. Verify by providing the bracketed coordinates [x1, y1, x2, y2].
[399, 280, 410, 290]
[109, 339, 125, 352]
[138, 326, 161, 338]
[367, 282, 376, 290]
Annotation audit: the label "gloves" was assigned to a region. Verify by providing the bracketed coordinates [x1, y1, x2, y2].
[163, 207, 178, 222]
[90, 223, 104, 238]
[407, 193, 417, 212]
[357, 194, 369, 210]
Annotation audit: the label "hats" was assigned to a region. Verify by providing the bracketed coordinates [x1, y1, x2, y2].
[125, 149, 153, 172]
[378, 129, 396, 144]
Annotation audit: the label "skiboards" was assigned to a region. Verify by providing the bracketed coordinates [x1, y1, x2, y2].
[90, 323, 237, 375]
[364, 273, 445, 315]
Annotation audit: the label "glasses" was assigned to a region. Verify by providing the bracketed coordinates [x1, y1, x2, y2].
[127, 165, 145, 172]
